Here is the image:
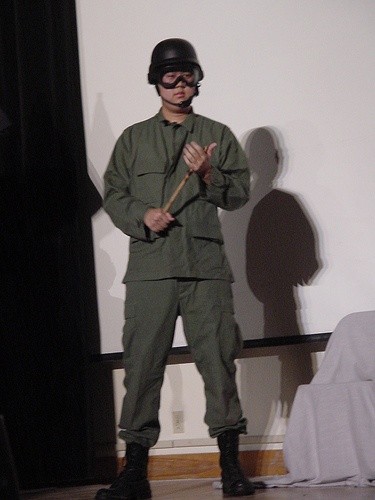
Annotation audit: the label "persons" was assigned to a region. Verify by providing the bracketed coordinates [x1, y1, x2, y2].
[94, 38, 257, 500]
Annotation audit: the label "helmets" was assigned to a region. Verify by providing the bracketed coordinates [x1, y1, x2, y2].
[148, 38, 203, 84]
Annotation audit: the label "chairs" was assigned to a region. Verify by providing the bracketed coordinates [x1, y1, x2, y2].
[282, 309, 375, 479]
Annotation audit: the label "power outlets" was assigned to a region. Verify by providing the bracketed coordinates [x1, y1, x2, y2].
[172, 410, 184, 434]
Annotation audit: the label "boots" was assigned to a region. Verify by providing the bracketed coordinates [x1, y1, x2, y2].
[95, 442, 151, 500]
[217, 430, 254, 496]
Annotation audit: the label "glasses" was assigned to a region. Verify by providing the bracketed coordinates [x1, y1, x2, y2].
[162, 70, 200, 88]
